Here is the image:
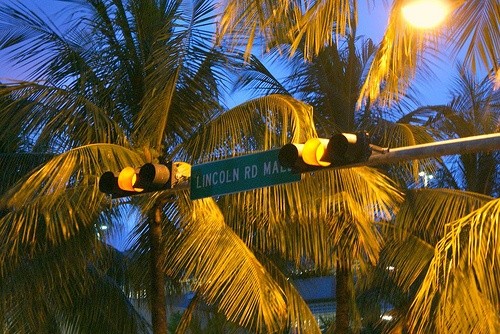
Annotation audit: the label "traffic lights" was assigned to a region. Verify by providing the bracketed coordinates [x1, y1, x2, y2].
[279, 131, 371, 172]
[99, 163, 170, 194]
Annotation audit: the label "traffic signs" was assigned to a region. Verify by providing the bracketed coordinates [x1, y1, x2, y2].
[190, 147, 301, 199]
